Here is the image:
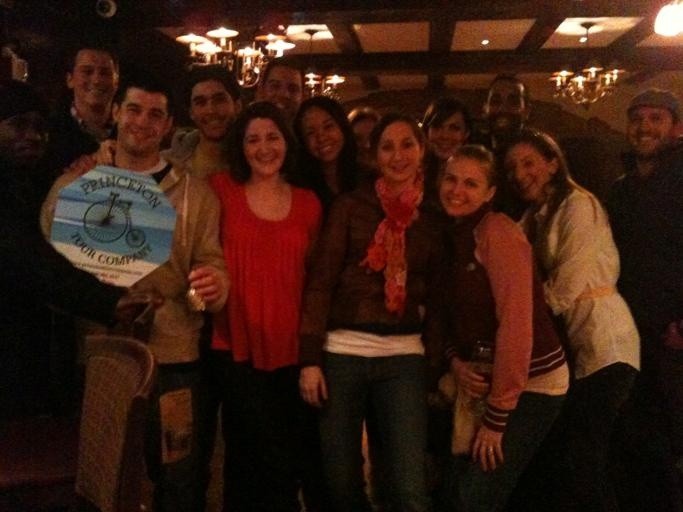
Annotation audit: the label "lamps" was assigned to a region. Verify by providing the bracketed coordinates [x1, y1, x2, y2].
[549, 22, 625, 110]
[304, 30, 346, 101]
[174, 22, 295, 88]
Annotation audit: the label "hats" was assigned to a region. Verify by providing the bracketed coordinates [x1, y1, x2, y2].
[626, 86, 682, 122]
[0, 77, 50, 121]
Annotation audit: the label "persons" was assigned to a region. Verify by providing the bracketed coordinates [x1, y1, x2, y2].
[2, 42, 683, 510]
